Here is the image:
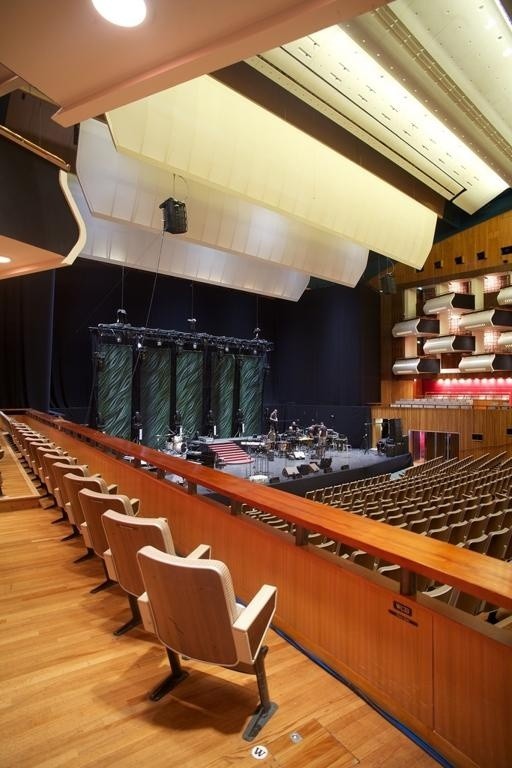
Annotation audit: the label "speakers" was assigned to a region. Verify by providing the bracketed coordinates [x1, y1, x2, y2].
[282, 462, 320, 477]
[159, 197, 188, 234]
[269, 477, 280, 483]
[324, 467, 332, 472]
[341, 464, 349, 470]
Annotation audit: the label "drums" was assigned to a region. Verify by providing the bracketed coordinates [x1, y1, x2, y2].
[176, 442, 186, 454]
[174, 435, 185, 442]
[278, 441, 290, 453]
[166, 442, 174, 451]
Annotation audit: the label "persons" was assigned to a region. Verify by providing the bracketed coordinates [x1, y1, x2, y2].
[284, 418, 327, 446]
[268, 408, 279, 432]
